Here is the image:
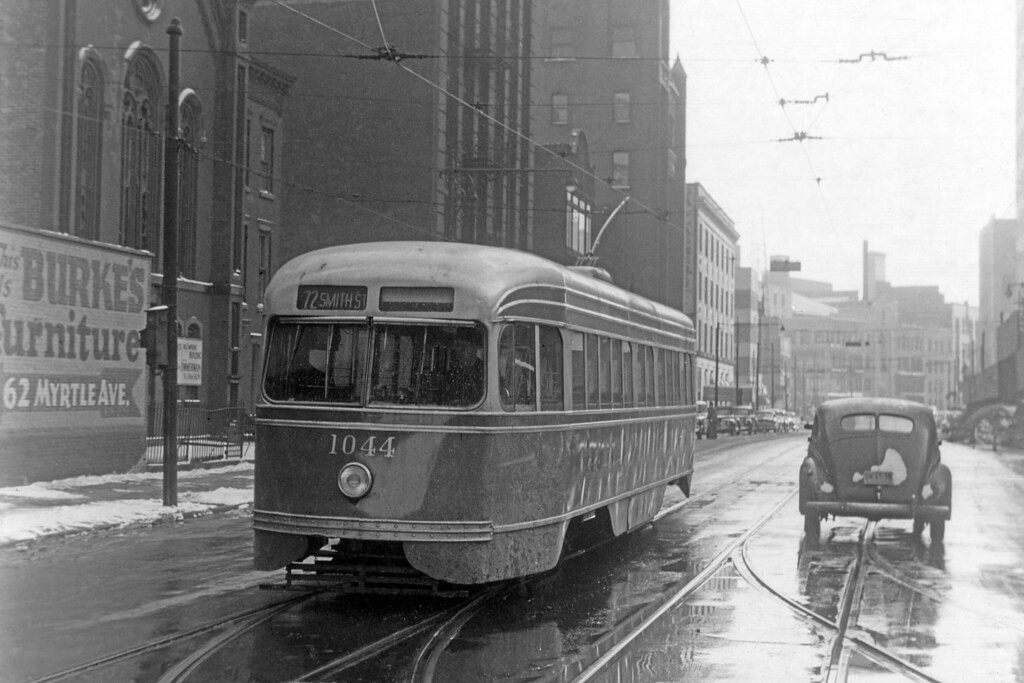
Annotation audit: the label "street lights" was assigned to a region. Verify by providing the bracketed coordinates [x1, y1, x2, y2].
[755, 323, 785, 406]
[1005, 283, 1023, 298]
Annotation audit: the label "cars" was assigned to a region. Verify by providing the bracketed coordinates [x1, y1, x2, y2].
[799, 398, 954, 541]
[698, 402, 709, 438]
[756, 410, 799, 433]
[715, 407, 737, 435]
[734, 405, 757, 434]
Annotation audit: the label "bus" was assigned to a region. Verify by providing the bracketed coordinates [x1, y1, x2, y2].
[253, 236, 697, 595]
